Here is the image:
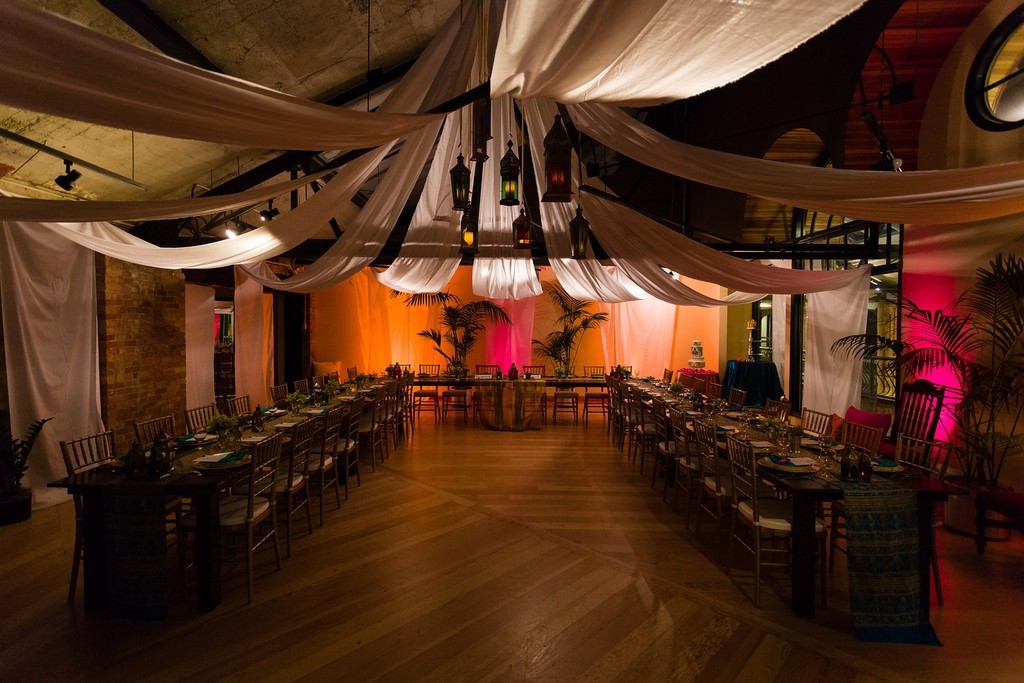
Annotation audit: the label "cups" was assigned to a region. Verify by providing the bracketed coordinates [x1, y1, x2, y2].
[776, 436, 790, 450]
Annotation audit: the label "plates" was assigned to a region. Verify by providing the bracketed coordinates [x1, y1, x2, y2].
[757, 457, 820, 473]
[274, 422, 297, 427]
[873, 465, 904, 472]
[193, 453, 252, 474]
[264, 409, 289, 415]
[801, 444, 845, 451]
[236, 436, 268, 441]
[716, 430, 740, 434]
[175, 434, 219, 445]
[725, 447, 778, 453]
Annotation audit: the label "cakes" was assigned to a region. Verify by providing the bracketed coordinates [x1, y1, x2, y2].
[688, 340, 705, 368]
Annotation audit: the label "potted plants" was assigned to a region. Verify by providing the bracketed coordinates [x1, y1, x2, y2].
[391, 289, 513, 406]
[532, 281, 609, 408]
[830, 252, 1023, 538]
[0, 416, 54, 525]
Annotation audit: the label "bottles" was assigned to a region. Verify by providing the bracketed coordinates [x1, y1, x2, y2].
[651, 382, 655, 392]
[508, 362, 518, 380]
[496, 366, 502, 380]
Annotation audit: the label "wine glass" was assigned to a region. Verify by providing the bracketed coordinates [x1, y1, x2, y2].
[314, 395, 322, 409]
[635, 369, 639, 382]
[661, 387, 666, 397]
[739, 407, 752, 440]
[818, 434, 836, 477]
[705, 399, 726, 420]
[194, 426, 207, 454]
[678, 389, 684, 405]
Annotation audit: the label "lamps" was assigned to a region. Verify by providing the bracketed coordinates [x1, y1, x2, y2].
[225, 215, 247, 238]
[511, 98, 534, 248]
[54, 159, 82, 191]
[568, 145, 590, 260]
[458, 209, 480, 254]
[499, 94, 520, 206]
[260, 199, 279, 222]
[540, 103, 574, 203]
[449, 108, 471, 211]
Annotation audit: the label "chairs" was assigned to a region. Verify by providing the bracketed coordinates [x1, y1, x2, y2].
[47, 361, 956, 618]
